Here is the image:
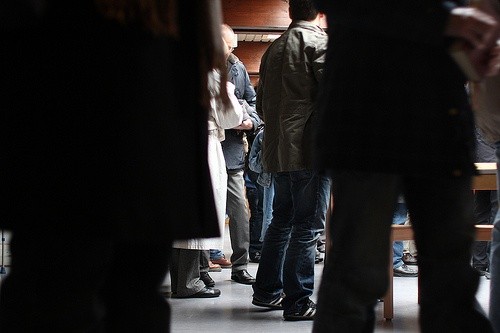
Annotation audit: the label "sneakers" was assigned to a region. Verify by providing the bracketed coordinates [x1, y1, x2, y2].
[252, 294, 286, 307]
[208, 261, 221, 272]
[251, 252, 260, 263]
[475, 265, 490, 276]
[212, 258, 232, 267]
[283, 300, 317, 320]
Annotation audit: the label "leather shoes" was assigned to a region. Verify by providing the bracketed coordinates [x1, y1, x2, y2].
[231, 270, 256, 284]
[403, 250, 417, 265]
[173, 288, 220, 298]
[393, 266, 418, 277]
[200, 273, 215, 285]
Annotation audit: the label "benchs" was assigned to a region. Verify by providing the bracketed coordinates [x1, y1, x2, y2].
[324, 161, 500, 330]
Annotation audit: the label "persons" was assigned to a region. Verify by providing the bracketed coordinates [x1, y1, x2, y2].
[171, 29, 498, 286]
[312, 0, 500, 333]
[250, 1, 335, 322]
[170, 44, 243, 299]
[198, 22, 260, 288]
